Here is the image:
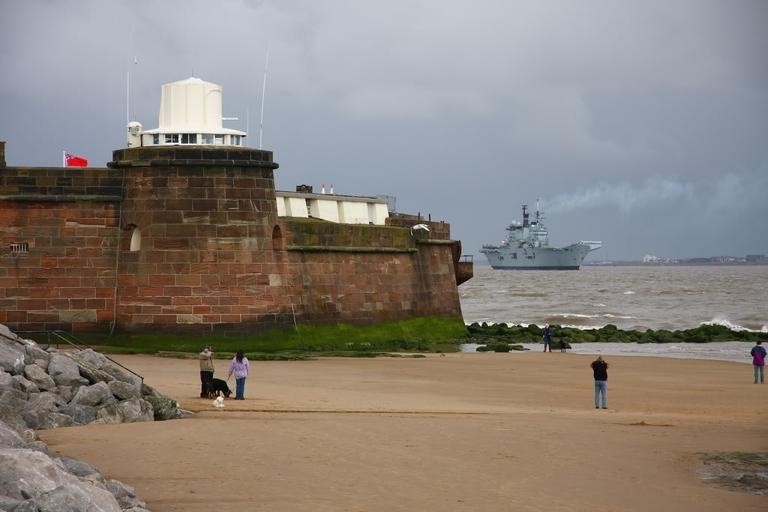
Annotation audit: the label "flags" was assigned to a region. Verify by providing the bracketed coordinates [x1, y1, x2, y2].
[63, 151, 89, 168]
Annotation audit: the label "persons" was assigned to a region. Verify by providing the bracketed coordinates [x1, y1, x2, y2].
[589, 355, 609, 409]
[227, 348, 250, 400]
[542, 322, 553, 353]
[750, 339, 768, 384]
[198, 344, 215, 398]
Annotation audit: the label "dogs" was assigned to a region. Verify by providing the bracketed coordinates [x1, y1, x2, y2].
[212, 396, 225, 408]
[206, 378, 232, 400]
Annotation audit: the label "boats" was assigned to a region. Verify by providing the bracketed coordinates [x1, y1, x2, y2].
[64, 55, 474, 286]
[479, 197, 603, 272]
